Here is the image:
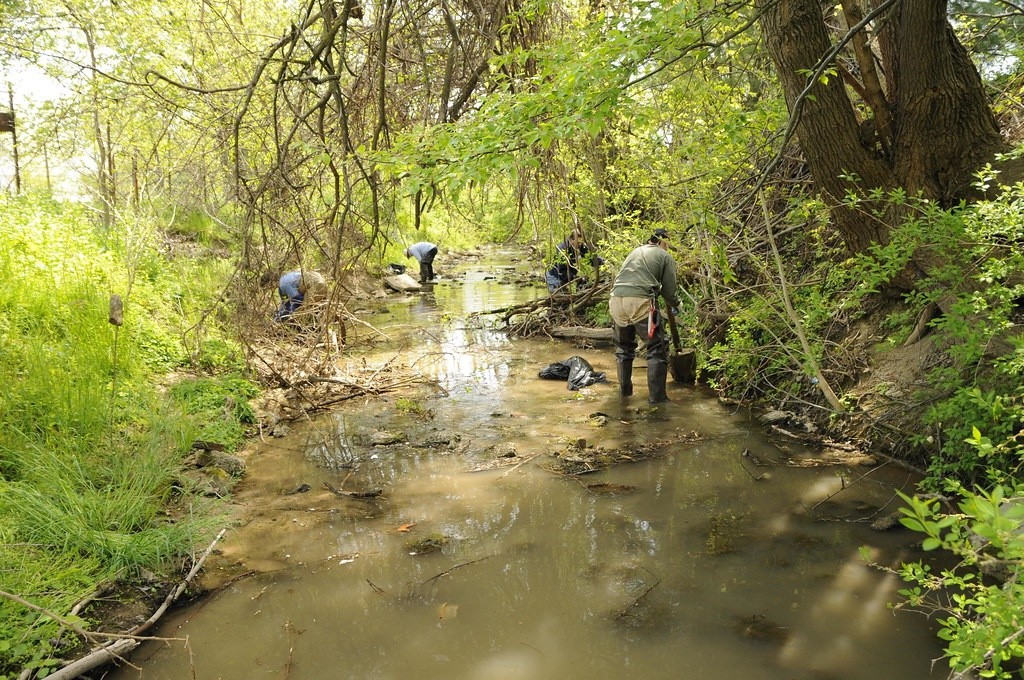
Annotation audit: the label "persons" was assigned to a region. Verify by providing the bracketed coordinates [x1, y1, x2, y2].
[261, 265, 325, 332]
[402, 242, 437, 282]
[609, 229, 676, 401]
[545, 229, 604, 294]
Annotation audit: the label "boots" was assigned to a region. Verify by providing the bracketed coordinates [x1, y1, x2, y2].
[645, 363, 670, 402]
[616, 360, 633, 397]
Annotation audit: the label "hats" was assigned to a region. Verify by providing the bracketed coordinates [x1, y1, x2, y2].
[403, 249, 408, 258]
